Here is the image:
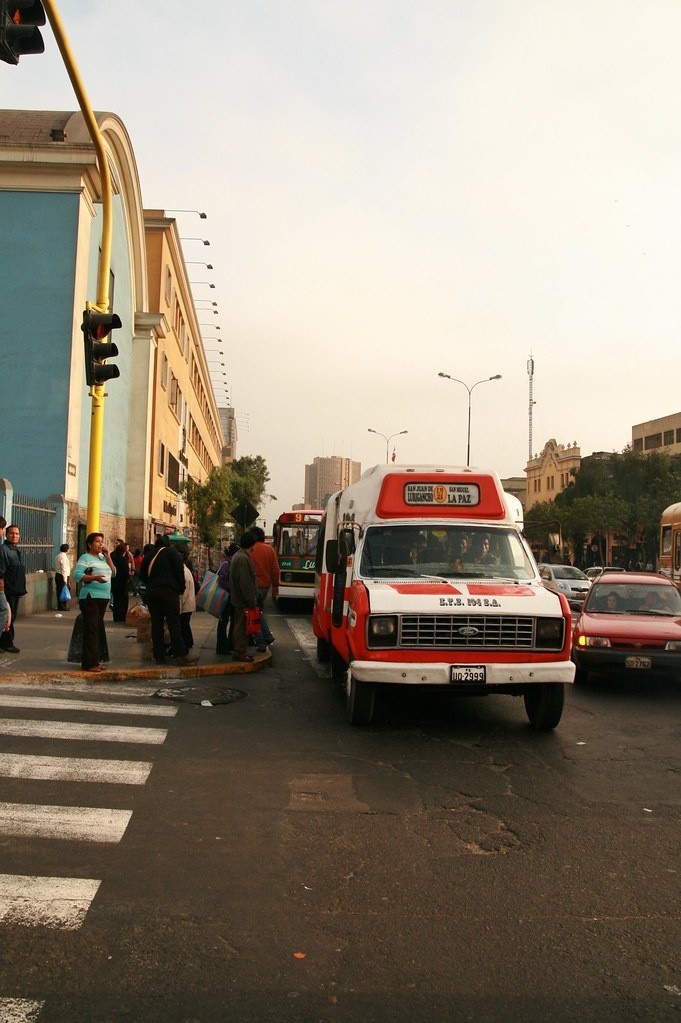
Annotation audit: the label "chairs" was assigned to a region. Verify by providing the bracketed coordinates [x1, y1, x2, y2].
[599, 595, 624, 612]
[418, 549, 450, 564]
[368, 545, 409, 566]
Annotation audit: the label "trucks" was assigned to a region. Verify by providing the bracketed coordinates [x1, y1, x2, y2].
[311, 465, 576, 729]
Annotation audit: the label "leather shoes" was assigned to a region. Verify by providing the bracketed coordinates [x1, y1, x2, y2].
[88, 664, 106, 672]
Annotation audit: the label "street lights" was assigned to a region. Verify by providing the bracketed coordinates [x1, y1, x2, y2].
[438, 372, 502, 465]
[368, 429, 408, 464]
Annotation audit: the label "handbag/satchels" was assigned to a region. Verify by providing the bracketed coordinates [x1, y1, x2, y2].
[195, 561, 230, 622]
[243, 607, 261, 633]
[60, 584, 71, 602]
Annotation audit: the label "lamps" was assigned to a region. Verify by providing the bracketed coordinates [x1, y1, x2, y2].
[163, 208, 250, 446]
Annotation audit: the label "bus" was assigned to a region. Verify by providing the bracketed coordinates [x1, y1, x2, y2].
[658, 501, 681, 593]
[272, 509, 324, 608]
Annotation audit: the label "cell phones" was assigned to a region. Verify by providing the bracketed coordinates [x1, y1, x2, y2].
[101, 546, 104, 553]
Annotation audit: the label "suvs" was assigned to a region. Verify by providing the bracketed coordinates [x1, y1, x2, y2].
[569, 570, 681, 686]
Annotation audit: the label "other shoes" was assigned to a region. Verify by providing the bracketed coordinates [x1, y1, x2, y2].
[109, 603, 113, 609]
[227, 650, 234, 655]
[58, 606, 70, 611]
[0, 646, 19, 653]
[232, 654, 254, 661]
[176, 653, 199, 666]
[255, 634, 275, 652]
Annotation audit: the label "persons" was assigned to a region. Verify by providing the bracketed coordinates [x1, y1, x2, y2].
[608, 591, 622, 610]
[67, 532, 117, 673]
[366, 532, 503, 566]
[0, 516, 28, 654]
[139, 534, 196, 664]
[110, 539, 144, 623]
[55, 544, 71, 611]
[542, 553, 586, 579]
[638, 591, 673, 612]
[621, 556, 654, 573]
[216, 527, 282, 662]
[664, 534, 680, 553]
[277, 529, 318, 555]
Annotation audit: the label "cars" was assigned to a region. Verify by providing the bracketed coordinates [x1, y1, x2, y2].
[538, 564, 593, 607]
[580, 567, 626, 584]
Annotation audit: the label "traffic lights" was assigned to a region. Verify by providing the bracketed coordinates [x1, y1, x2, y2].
[0, 0, 46, 65]
[81, 310, 120, 386]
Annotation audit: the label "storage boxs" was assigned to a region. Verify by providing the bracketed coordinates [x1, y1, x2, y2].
[136, 617, 153, 642]
[126, 603, 150, 626]
[142, 629, 172, 659]
